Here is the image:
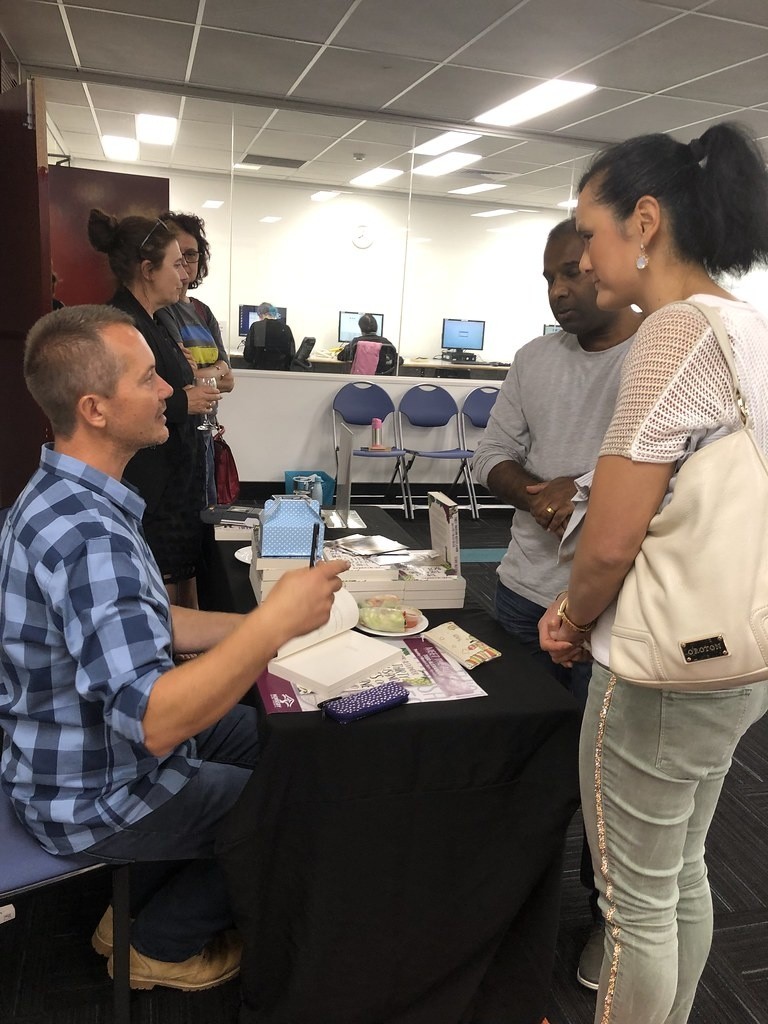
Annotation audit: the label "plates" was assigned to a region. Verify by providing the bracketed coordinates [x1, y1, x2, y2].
[234, 545, 253, 564]
[355, 614, 429, 637]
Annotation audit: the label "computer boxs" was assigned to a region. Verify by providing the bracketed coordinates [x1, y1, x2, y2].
[442, 352, 476, 362]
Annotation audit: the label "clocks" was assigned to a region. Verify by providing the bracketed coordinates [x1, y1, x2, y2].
[352, 225, 374, 250]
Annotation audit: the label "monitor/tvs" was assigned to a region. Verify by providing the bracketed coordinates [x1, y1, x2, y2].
[442, 319, 485, 355]
[239, 304, 287, 336]
[543, 324, 563, 336]
[338, 312, 384, 343]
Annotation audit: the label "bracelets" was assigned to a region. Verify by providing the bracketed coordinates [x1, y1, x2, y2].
[212, 365, 224, 381]
[556, 597, 593, 636]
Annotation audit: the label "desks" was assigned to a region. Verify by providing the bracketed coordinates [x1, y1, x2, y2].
[226, 351, 511, 371]
[210, 506, 584, 1024]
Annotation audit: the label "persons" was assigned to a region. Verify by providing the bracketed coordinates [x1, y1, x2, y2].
[470, 212, 644, 991]
[0, 306, 352, 993]
[243, 302, 295, 371]
[82, 207, 234, 614]
[537, 121, 768, 1024]
[52, 273, 64, 311]
[337, 314, 404, 376]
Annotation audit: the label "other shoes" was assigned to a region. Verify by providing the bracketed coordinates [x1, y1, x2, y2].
[577, 930, 605, 990]
[92, 904, 135, 958]
[107, 937, 243, 991]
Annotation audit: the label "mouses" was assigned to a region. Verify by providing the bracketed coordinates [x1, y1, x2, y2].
[490, 362, 498, 366]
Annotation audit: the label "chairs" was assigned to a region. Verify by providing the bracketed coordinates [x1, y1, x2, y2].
[351, 341, 404, 375]
[289, 337, 316, 371]
[0, 788, 131, 1024]
[332, 380, 516, 521]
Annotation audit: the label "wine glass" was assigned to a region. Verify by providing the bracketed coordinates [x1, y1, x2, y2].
[192, 376, 217, 430]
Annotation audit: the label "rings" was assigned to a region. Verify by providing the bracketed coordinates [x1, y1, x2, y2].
[209, 402, 211, 408]
[546, 506, 556, 515]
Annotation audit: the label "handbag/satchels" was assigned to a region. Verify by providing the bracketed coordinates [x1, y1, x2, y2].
[211, 425, 240, 504]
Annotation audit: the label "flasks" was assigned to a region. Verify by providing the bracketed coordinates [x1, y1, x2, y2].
[372, 418, 382, 447]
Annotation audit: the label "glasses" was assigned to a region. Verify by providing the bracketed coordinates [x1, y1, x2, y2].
[138, 218, 168, 248]
[182, 248, 202, 263]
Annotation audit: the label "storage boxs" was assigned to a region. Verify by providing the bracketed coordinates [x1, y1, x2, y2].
[258, 471, 335, 557]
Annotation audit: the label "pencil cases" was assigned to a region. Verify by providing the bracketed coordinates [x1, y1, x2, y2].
[317, 681, 409, 725]
[420, 622, 501, 670]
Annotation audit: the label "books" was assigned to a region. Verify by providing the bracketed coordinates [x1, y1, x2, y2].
[249, 491, 466, 610]
[266, 587, 403, 699]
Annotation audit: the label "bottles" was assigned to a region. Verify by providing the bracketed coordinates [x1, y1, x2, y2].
[312, 476, 323, 506]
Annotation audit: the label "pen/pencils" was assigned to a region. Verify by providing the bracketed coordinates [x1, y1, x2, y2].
[310, 523, 320, 567]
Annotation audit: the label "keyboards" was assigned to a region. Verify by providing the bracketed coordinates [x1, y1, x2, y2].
[452, 361, 490, 366]
